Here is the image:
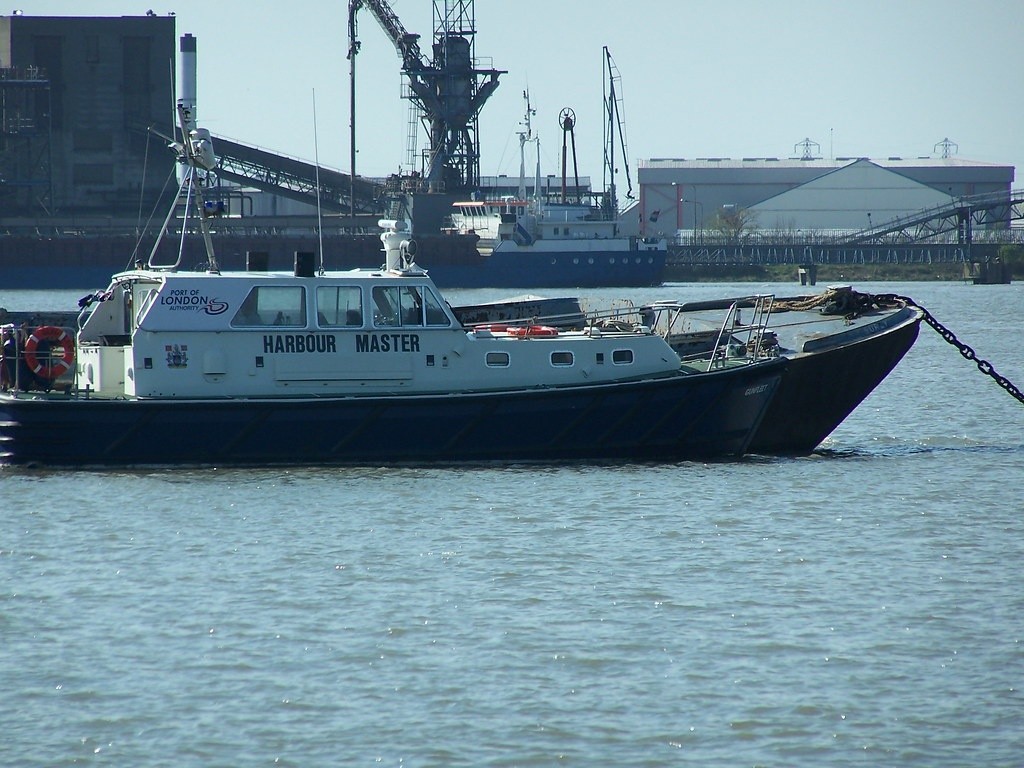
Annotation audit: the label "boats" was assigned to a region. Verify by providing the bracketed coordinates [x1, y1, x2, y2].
[0, 101, 922, 466]
[378, 0, 667, 288]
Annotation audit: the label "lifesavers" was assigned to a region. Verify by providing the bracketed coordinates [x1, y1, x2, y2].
[507, 327, 559, 338]
[26, 326, 74, 377]
[473, 324, 509, 332]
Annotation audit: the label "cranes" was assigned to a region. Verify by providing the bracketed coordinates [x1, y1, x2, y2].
[346, 0, 509, 237]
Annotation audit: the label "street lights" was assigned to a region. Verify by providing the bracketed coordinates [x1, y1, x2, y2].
[671, 182, 706, 263]
